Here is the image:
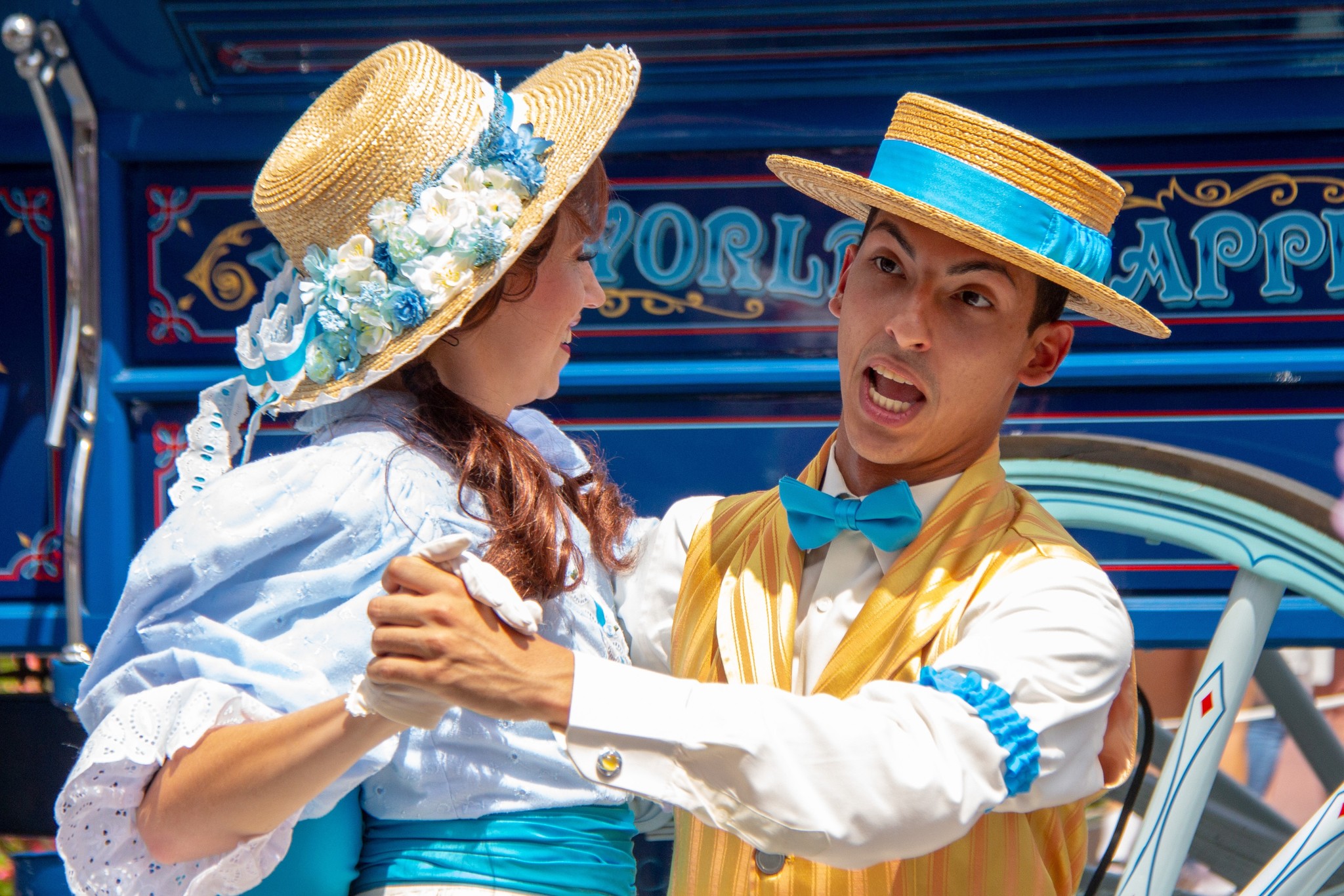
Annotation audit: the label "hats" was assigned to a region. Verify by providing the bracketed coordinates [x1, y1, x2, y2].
[767, 92, 1174, 341]
[233, 41, 643, 414]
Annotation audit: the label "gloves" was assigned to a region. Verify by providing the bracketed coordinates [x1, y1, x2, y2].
[343, 533, 542, 731]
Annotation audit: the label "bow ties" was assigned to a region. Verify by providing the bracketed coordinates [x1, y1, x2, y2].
[778, 476, 921, 554]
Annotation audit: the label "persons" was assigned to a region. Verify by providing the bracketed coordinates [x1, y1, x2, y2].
[53, 39, 660, 896]
[365, 92, 1172, 896]
